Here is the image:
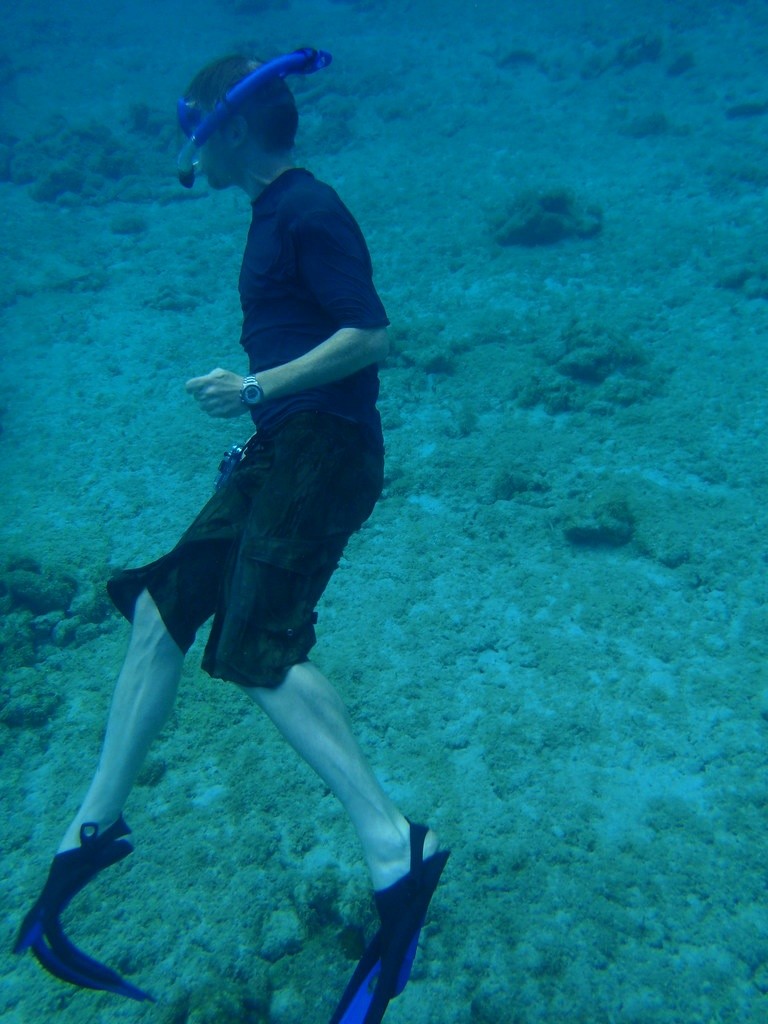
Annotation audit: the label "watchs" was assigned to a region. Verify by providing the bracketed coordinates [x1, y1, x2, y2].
[240, 373, 264, 410]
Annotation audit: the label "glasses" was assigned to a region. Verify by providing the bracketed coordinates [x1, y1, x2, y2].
[176, 97, 203, 138]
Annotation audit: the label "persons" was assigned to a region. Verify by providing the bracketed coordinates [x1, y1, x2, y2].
[10, 46, 452, 1024]
